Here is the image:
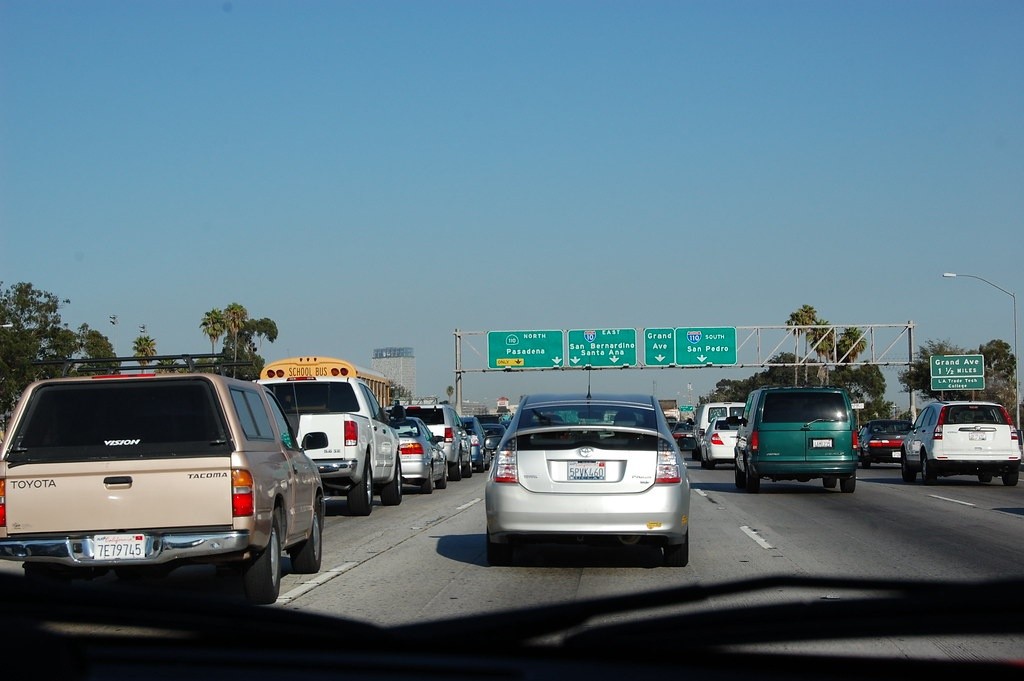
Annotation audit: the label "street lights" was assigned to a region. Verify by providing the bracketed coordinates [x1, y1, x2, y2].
[941, 271, 1021, 430]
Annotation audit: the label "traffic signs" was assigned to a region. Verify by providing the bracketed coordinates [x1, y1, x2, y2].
[644, 325, 737, 367]
[488, 329, 564, 372]
[568, 328, 638, 367]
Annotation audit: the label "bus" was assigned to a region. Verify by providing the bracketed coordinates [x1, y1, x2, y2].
[260, 355, 393, 409]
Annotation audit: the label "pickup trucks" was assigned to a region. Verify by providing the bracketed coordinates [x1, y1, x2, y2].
[249, 373, 407, 517]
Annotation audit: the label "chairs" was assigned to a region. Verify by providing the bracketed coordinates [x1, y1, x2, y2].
[602, 412, 639, 439]
[533, 414, 569, 440]
[972, 415, 994, 423]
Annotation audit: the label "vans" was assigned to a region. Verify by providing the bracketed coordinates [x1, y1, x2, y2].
[692, 401, 746, 462]
[726, 384, 859, 493]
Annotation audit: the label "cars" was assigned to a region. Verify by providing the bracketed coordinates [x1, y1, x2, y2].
[698, 415, 742, 470]
[390, 415, 449, 494]
[660, 400, 694, 452]
[857, 419, 915, 469]
[484, 393, 700, 569]
[459, 416, 492, 472]
[478, 414, 514, 453]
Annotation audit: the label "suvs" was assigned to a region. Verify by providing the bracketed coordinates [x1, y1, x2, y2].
[385, 403, 473, 481]
[0, 352, 329, 607]
[900, 398, 1023, 486]
